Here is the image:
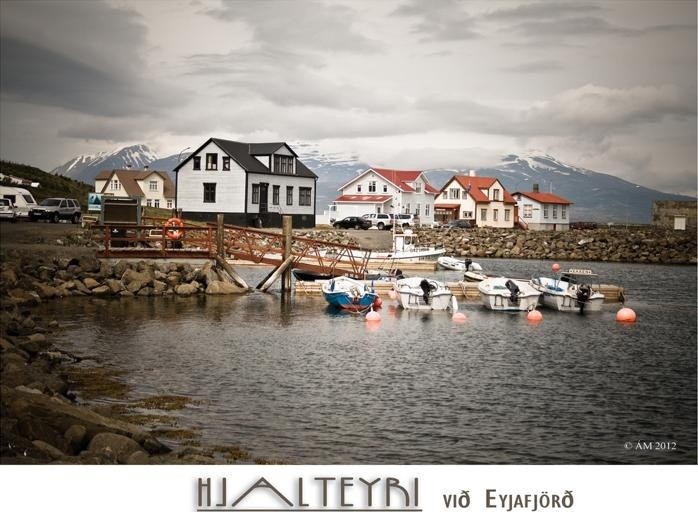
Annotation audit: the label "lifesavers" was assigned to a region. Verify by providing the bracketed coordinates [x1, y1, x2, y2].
[165, 218, 184, 239]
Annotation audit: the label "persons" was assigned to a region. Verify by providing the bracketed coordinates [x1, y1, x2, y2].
[93, 194, 100, 204]
[170, 239, 184, 249]
[89, 196, 94, 204]
[419, 279, 431, 305]
[465, 254, 473, 273]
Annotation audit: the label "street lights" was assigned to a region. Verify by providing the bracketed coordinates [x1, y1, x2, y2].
[177, 147, 191, 163]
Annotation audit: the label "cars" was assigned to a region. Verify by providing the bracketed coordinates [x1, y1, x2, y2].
[0, 198, 81, 223]
[333, 213, 420, 230]
[149, 229, 174, 238]
[454, 219, 476, 228]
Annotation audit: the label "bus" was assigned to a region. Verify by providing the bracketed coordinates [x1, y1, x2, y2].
[569, 222, 598, 230]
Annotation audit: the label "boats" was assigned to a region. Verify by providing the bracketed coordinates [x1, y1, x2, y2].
[438, 256, 483, 271]
[307, 234, 446, 261]
